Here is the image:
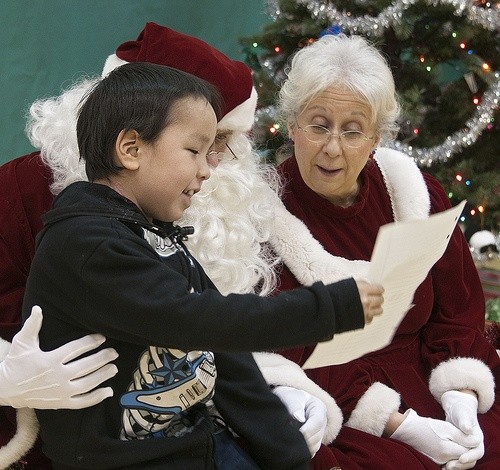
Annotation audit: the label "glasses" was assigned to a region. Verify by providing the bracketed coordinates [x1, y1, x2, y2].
[205, 143, 239, 161]
[480, 245, 497, 253]
[294, 111, 374, 149]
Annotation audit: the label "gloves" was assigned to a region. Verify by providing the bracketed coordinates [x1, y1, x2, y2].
[273, 385, 327, 459]
[440, 390, 484, 470]
[0, 305, 119, 410]
[389, 408, 481, 465]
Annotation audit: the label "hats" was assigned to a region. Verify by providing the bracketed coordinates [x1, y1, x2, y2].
[469, 231, 497, 253]
[100, 20, 258, 134]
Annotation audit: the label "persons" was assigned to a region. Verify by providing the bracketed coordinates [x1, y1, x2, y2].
[255, 33, 500, 470]
[0, 22, 440, 470]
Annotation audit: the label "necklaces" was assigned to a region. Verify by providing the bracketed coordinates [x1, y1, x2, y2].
[21, 63, 386, 470]
